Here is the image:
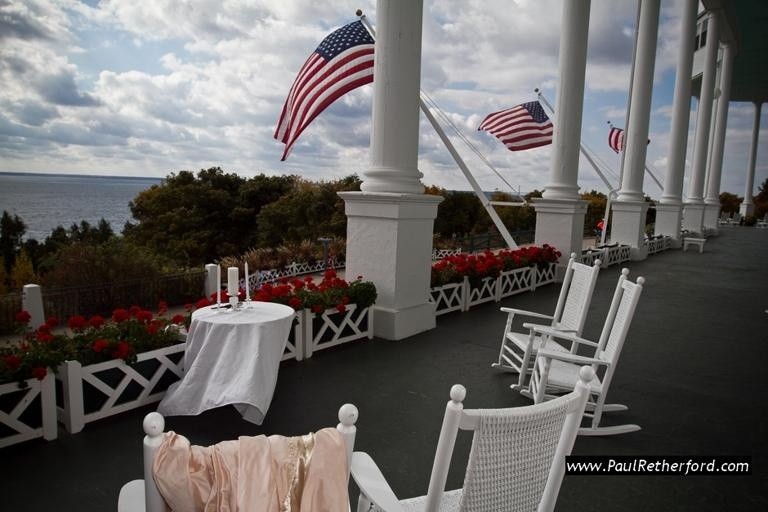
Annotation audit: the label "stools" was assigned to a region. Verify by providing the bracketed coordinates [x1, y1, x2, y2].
[684, 237, 706, 252]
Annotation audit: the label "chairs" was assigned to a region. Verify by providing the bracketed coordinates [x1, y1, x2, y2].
[492, 253, 602, 391]
[349, 367, 594, 512]
[520, 268, 645, 437]
[117, 404, 359, 512]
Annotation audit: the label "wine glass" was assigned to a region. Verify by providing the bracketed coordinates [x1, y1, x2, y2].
[229, 296, 240, 313]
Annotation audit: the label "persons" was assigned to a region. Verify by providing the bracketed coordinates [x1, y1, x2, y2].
[596, 218, 607, 244]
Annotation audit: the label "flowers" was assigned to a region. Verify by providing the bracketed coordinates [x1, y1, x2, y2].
[0, 245, 560, 388]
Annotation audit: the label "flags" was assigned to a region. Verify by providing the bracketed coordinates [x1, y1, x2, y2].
[274, 20, 374, 165]
[606, 126, 651, 155]
[476, 99, 555, 153]
